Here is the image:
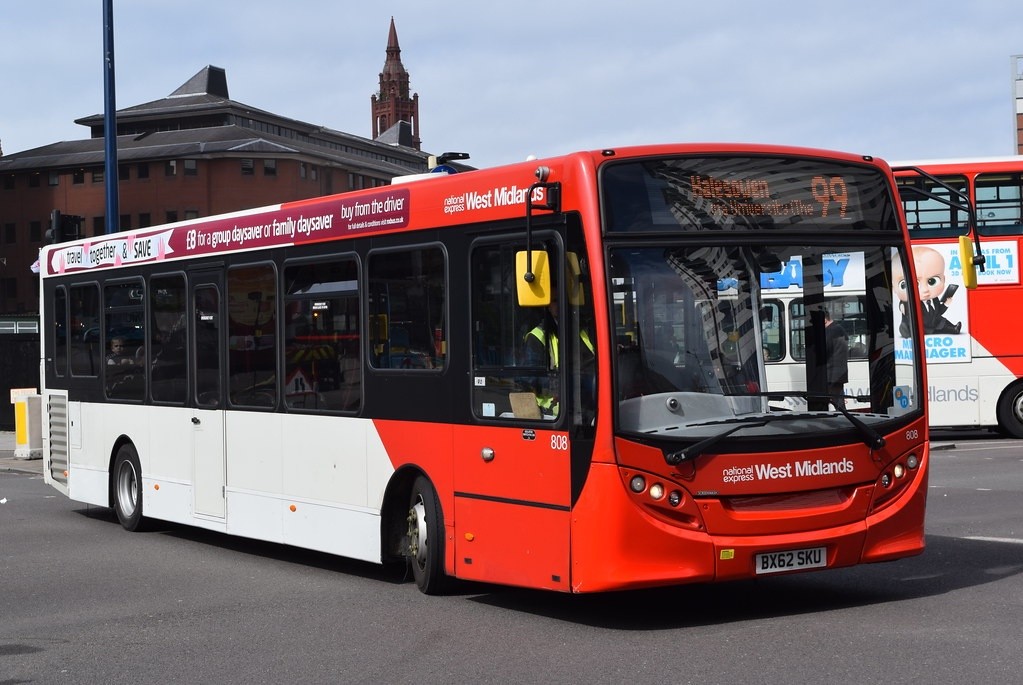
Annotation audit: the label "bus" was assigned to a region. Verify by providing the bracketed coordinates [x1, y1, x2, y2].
[31, 143, 987, 597]
[612, 158, 1023, 435]
[289, 281, 377, 345]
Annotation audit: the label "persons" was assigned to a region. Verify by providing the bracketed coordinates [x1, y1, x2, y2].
[824, 306, 849, 411]
[523, 284, 596, 427]
[106, 336, 134, 367]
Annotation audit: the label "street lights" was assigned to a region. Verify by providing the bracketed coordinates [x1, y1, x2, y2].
[428, 151, 470, 171]
